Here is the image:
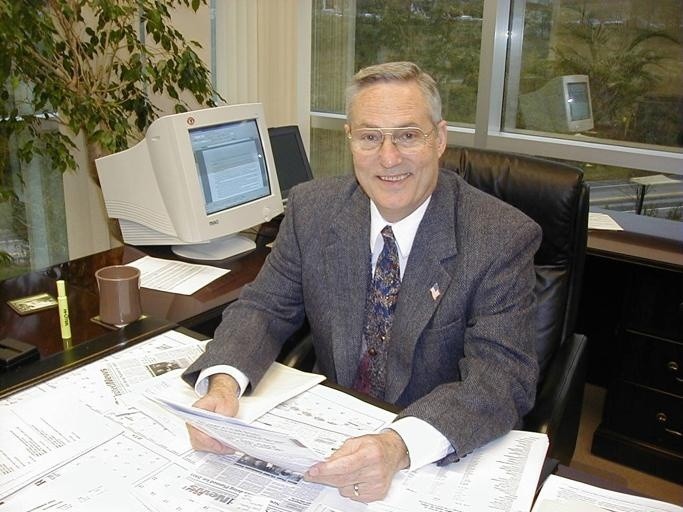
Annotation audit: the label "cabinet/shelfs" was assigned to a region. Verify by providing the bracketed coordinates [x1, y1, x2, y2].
[584, 226, 683, 486]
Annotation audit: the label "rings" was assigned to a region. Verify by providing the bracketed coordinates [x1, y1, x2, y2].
[353, 484, 359, 497]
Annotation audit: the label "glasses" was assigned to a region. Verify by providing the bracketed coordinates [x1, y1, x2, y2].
[349, 127, 436, 154]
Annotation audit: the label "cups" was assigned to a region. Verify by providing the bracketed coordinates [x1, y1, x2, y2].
[95, 265, 141, 323]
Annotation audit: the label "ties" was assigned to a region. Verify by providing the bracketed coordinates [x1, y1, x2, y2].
[364, 225, 401, 401]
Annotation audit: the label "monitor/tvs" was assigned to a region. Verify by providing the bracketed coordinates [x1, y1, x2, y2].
[518, 73, 594, 137]
[93, 102, 284, 260]
[268, 124, 315, 208]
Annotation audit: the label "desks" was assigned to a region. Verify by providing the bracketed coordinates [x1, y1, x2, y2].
[2, 226, 277, 396]
[2, 324, 683, 511]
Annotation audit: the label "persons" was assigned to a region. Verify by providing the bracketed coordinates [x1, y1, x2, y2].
[178, 61, 545, 506]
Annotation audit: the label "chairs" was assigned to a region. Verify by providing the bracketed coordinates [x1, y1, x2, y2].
[275, 146, 590, 458]
